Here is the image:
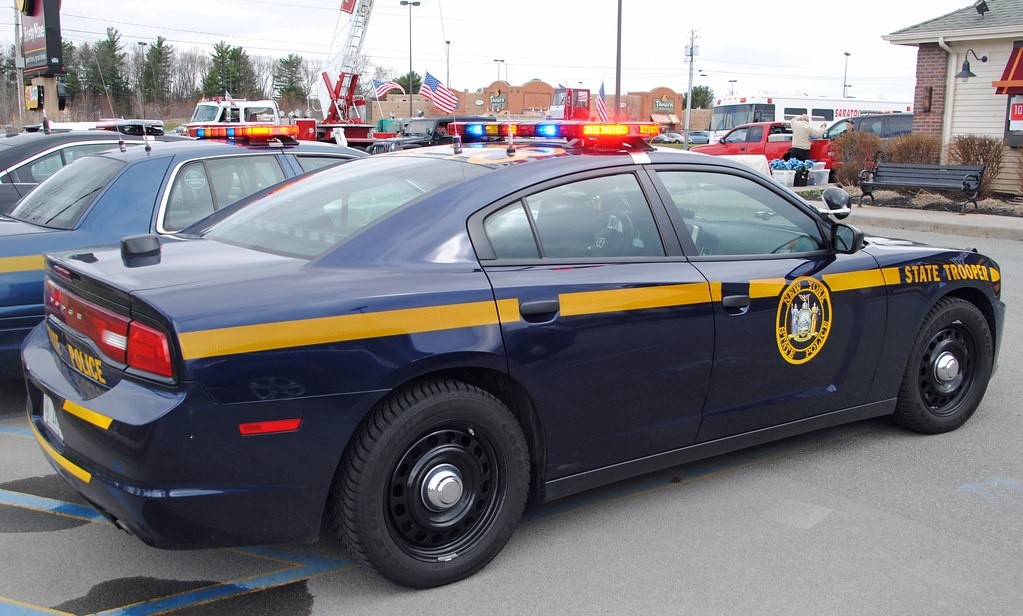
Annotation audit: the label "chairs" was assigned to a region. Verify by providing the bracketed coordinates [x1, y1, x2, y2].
[534, 192, 594, 259]
[194, 167, 235, 216]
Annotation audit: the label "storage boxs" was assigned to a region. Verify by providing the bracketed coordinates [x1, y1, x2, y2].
[773, 170, 796, 187]
[806, 162, 830, 186]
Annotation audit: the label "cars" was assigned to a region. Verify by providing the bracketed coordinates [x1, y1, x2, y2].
[0, 110, 431, 381]
[19, 117, 1010, 591]
[643, 129, 711, 145]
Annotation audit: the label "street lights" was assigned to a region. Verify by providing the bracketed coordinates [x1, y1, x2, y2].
[729, 79, 738, 98]
[445, 41, 451, 92]
[399, 1, 421, 120]
[494, 58, 505, 94]
[841, 52, 852, 100]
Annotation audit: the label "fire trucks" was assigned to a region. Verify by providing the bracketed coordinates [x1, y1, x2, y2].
[170, 1, 399, 154]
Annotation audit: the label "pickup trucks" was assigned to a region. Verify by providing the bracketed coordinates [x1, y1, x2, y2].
[684, 120, 876, 183]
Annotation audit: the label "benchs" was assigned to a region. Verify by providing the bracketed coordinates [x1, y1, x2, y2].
[857, 161, 986, 216]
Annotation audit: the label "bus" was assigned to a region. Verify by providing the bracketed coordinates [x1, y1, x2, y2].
[705, 95, 914, 145]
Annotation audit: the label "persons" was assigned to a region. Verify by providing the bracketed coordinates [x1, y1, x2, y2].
[789, 114, 821, 162]
[839, 119, 855, 135]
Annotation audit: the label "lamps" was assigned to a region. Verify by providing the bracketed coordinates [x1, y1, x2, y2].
[955, 49, 987, 82]
[974, 0, 990, 16]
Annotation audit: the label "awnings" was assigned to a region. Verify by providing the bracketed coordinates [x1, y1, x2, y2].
[651, 114, 680, 125]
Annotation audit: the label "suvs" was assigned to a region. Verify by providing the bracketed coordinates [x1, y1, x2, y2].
[366, 116, 499, 160]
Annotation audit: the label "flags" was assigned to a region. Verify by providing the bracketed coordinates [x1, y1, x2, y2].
[417, 72, 458, 114]
[372, 80, 405, 97]
[226, 91, 235, 105]
[595, 82, 607, 122]
